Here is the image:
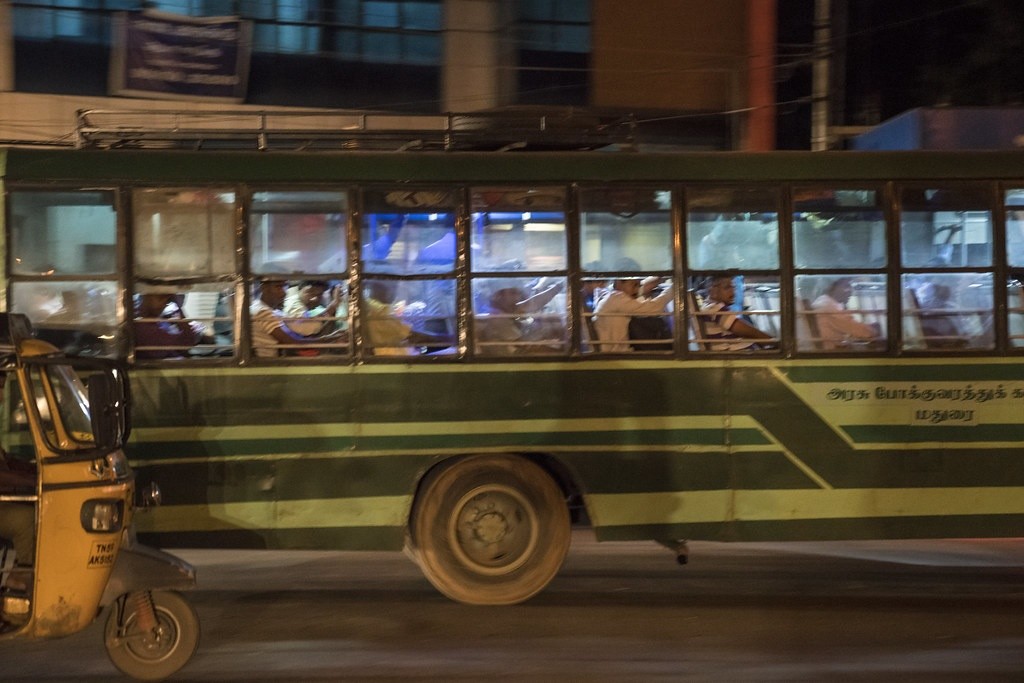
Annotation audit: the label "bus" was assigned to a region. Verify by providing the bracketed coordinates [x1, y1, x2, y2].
[0, 111, 1024, 606]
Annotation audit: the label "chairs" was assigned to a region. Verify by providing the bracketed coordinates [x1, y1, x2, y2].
[685, 289, 711, 352]
[846, 282, 932, 352]
[740, 284, 825, 352]
[554, 292, 599, 355]
[959, 283, 1024, 346]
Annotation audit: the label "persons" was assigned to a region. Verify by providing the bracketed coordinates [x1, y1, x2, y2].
[810, 262, 883, 353]
[357, 258, 572, 356]
[912, 256, 970, 347]
[0, 369, 34, 597]
[580, 257, 675, 353]
[246, 263, 349, 357]
[693, 257, 780, 351]
[131, 282, 235, 360]
[416, 216, 486, 264]
[362, 214, 408, 262]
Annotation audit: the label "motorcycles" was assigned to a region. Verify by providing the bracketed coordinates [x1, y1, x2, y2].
[0, 339, 200, 683]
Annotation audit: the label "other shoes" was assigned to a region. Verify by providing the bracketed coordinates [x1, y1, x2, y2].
[6, 565, 32, 590]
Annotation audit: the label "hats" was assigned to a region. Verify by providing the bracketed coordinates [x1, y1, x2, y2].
[486, 278, 523, 298]
[819, 273, 858, 290]
